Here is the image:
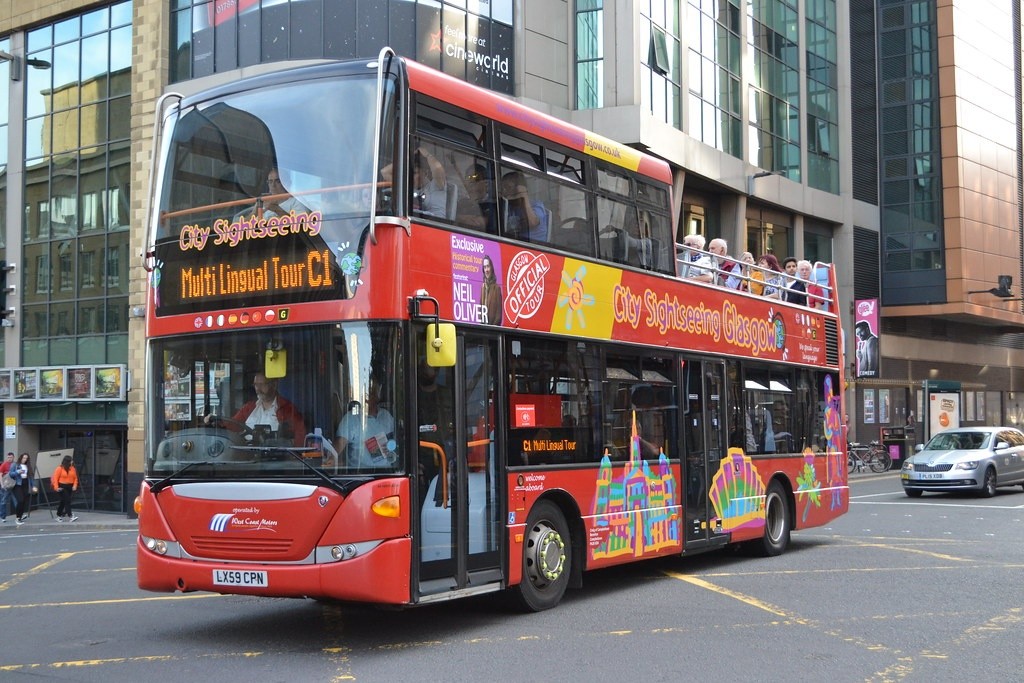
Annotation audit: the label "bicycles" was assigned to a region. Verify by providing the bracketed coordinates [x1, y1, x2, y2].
[846, 441, 892, 474]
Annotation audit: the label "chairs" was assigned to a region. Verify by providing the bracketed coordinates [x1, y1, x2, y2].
[447, 180, 831, 310]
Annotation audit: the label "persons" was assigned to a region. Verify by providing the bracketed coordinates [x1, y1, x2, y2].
[605, 207, 654, 270]
[322, 354, 447, 476]
[946, 434, 961, 449]
[845, 414, 851, 443]
[680, 234, 825, 310]
[244, 168, 308, 222]
[715, 386, 775, 455]
[855, 320, 880, 378]
[0, 452, 38, 525]
[205, 369, 309, 458]
[51, 455, 78, 523]
[360, 144, 548, 243]
[509, 414, 661, 465]
[478, 256, 503, 325]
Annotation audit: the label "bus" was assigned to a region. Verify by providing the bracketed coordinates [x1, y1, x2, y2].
[133, 46, 849, 613]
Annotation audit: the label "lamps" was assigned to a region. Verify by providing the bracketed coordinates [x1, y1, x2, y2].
[969, 275, 1014, 297]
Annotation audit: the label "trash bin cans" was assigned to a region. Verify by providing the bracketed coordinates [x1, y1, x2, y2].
[880, 427, 917, 470]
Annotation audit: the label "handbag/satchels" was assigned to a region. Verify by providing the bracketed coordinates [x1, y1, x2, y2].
[3, 462, 18, 489]
[50, 466, 62, 486]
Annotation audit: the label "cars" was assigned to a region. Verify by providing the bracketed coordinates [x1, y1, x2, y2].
[900, 426, 1024, 499]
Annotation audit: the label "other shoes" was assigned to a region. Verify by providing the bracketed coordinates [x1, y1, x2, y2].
[2, 519, 6, 523]
[55, 515, 63, 523]
[69, 516, 79, 522]
[21, 514, 27, 519]
[15, 518, 25, 525]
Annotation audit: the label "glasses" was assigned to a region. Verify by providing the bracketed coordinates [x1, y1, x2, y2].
[251, 380, 273, 388]
[684, 242, 697, 247]
